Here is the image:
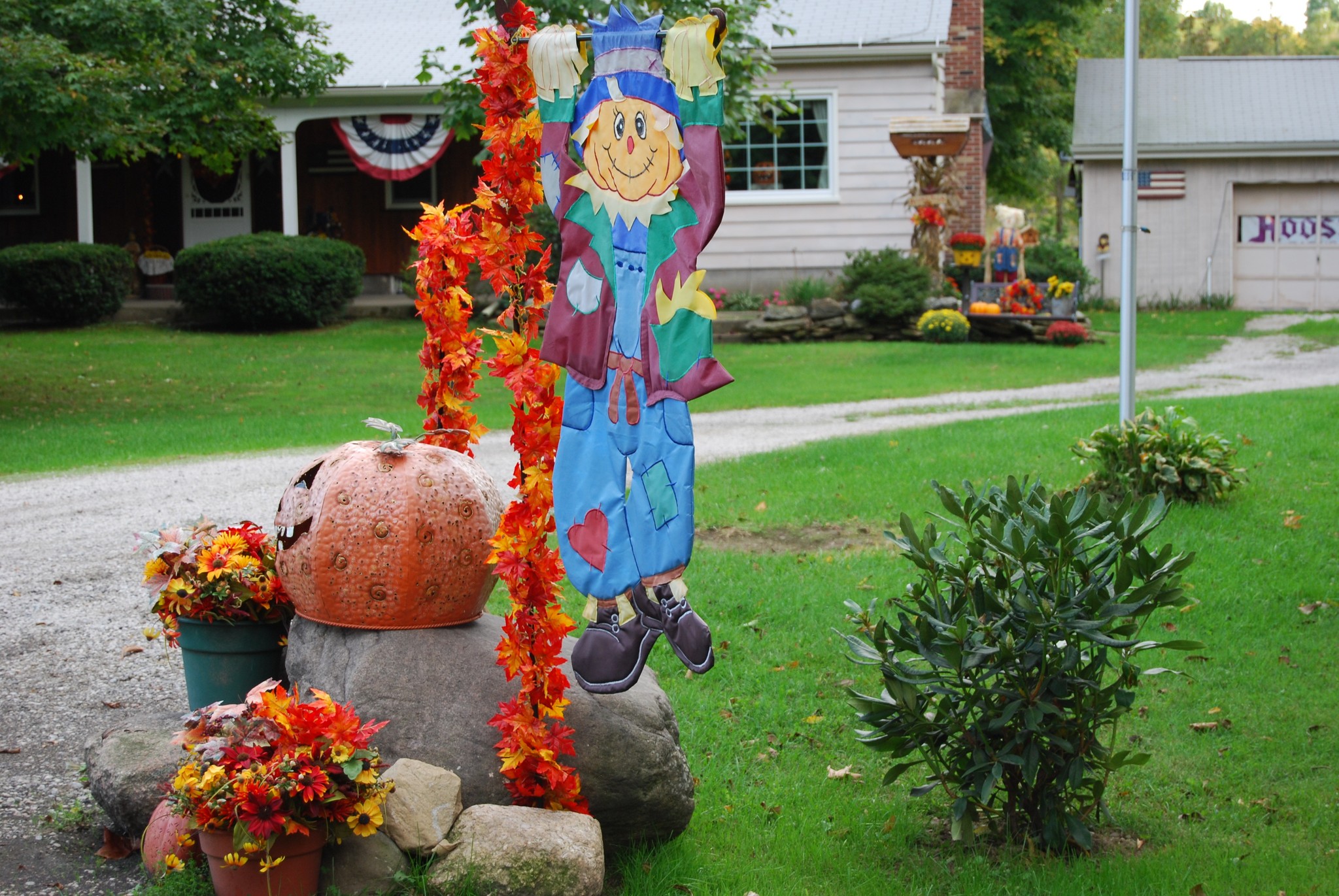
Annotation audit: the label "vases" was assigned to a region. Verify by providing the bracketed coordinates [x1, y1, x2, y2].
[176, 618, 287, 712]
[196, 824, 331, 896]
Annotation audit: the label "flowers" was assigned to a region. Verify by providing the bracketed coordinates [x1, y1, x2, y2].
[131, 512, 290, 669]
[140, 678, 396, 895]
[910, 201, 1091, 347]
